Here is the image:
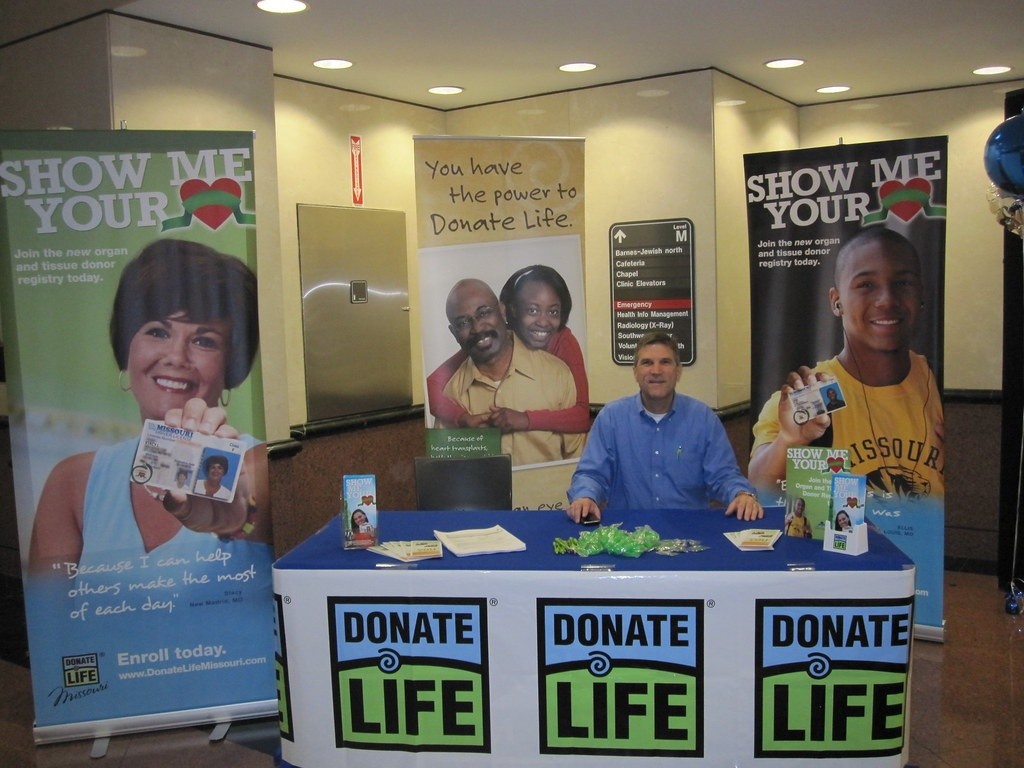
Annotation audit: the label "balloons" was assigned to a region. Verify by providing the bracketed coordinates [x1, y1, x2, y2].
[983, 113, 1024, 239]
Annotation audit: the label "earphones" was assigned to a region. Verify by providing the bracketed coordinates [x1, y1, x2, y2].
[836, 301, 841, 308]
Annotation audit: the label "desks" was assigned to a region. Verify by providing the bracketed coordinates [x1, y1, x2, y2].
[272, 507, 917, 768]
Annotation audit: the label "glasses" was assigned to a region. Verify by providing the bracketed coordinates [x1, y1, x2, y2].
[454, 300, 498, 330]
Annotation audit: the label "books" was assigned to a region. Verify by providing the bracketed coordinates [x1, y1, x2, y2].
[366, 525, 527, 563]
[723, 529, 783, 551]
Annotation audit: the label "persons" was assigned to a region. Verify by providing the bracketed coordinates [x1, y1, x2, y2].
[747, 226, 944, 538]
[426, 264, 591, 468]
[565, 333, 764, 523]
[350, 509, 375, 547]
[30, 237, 272, 613]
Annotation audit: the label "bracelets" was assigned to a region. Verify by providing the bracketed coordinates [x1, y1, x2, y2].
[211, 496, 257, 544]
[736, 492, 756, 497]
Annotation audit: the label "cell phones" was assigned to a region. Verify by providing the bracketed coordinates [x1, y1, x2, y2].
[580, 512, 600, 526]
